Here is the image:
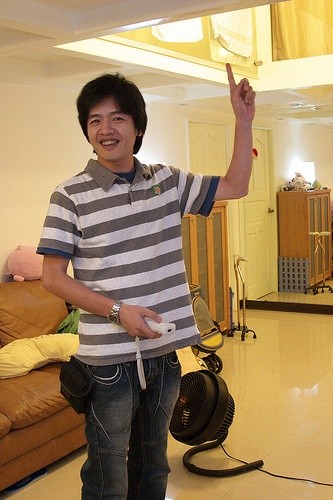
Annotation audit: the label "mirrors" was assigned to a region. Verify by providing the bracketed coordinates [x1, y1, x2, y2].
[238, 105, 333, 306]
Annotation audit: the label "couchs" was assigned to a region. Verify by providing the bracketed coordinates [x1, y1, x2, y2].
[0, 279, 89, 491]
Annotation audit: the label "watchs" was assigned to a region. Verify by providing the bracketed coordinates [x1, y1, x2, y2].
[107, 300, 123, 323]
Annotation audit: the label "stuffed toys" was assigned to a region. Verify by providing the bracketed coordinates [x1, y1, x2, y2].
[6, 245, 43, 282]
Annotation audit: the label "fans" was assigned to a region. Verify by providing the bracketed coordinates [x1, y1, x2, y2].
[169, 370, 264, 477]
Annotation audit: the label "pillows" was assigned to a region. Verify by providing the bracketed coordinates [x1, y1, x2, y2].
[0, 333, 80, 379]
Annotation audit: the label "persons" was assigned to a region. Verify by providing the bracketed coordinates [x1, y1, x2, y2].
[36, 63, 255, 500]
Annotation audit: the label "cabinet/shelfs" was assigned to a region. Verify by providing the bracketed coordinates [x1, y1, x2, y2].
[278, 189, 332, 286]
[181, 200, 229, 334]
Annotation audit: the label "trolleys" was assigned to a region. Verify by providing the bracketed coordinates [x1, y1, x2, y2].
[188, 284, 225, 375]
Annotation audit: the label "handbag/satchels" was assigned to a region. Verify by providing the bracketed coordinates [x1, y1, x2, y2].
[59, 354, 96, 416]
